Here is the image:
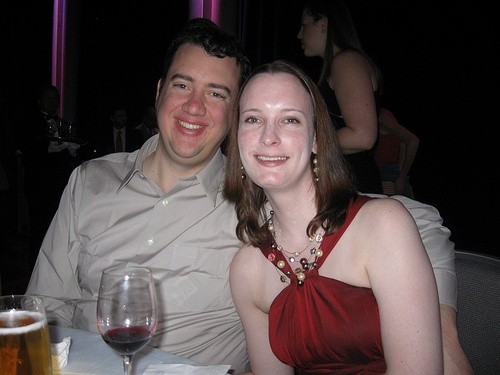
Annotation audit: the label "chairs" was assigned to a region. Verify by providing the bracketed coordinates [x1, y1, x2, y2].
[454, 249, 500, 375]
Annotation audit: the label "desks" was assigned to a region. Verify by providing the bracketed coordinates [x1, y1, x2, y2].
[48, 324, 233, 375]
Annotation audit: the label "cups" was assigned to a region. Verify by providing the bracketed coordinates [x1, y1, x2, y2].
[0, 295, 52, 375]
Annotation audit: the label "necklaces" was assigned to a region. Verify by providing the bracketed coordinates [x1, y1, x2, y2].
[266, 209, 330, 287]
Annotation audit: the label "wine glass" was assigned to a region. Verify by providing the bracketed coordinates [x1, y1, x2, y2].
[96, 266, 158, 375]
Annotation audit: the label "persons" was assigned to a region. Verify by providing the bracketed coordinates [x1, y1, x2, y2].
[1, 84, 158, 267]
[23, 16, 472, 375]
[226, 60, 444, 375]
[298, 0, 419, 196]
